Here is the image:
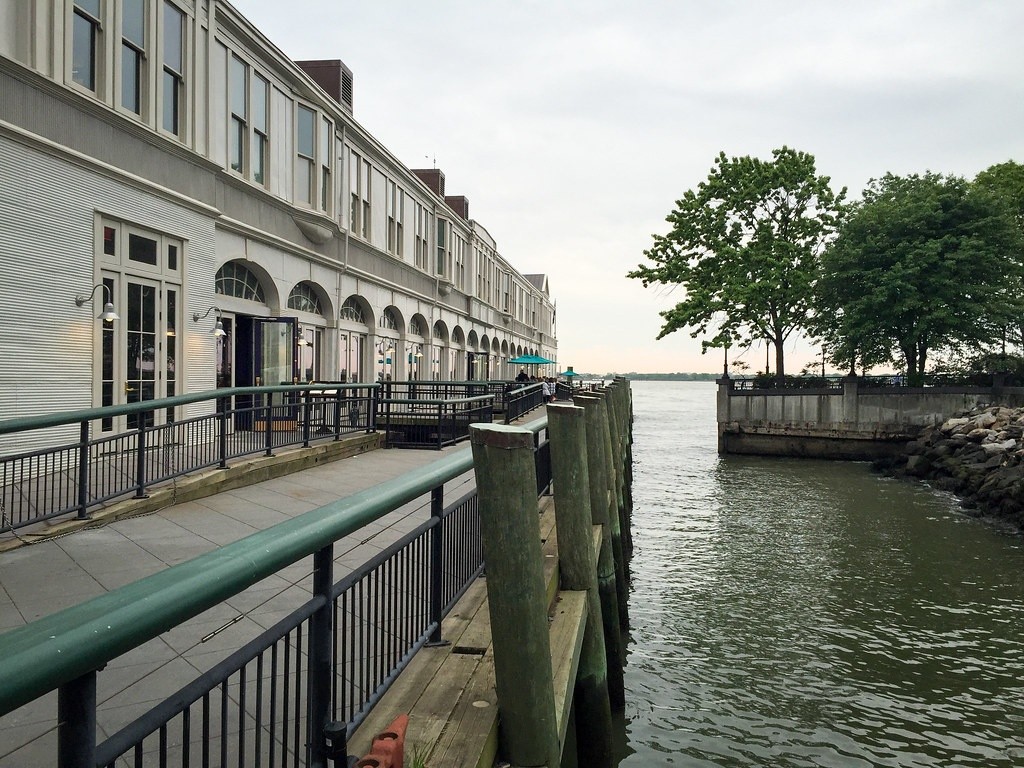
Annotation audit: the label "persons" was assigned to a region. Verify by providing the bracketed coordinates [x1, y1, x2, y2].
[517, 369, 529, 381]
[515, 376, 557, 403]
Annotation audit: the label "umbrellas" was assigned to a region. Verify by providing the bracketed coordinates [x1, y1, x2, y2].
[383, 358, 391, 364]
[408, 353, 417, 363]
[559, 369, 580, 382]
[507, 353, 557, 377]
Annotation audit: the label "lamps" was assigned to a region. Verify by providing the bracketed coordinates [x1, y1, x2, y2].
[465, 353, 551, 372]
[406, 342, 423, 357]
[75, 284, 121, 322]
[193, 306, 226, 336]
[375, 336, 395, 354]
[282, 322, 308, 346]
